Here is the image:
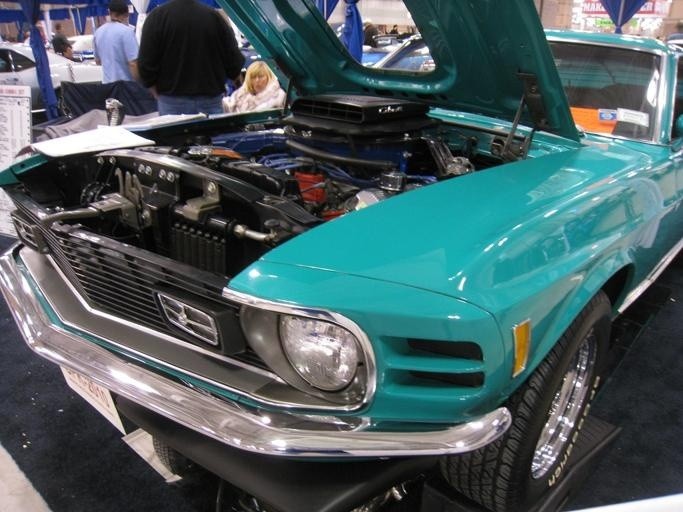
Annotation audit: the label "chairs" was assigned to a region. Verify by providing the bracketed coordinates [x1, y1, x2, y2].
[561, 82, 651, 135]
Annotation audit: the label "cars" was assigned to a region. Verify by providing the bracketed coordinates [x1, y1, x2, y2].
[0, 2, 682, 511]
[0, 41, 104, 117]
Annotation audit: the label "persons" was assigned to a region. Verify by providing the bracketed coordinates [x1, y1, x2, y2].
[92, 1, 140, 86]
[60, 42, 81, 62]
[220, 62, 287, 113]
[390, 25, 398, 34]
[360, 19, 378, 49]
[238, 34, 253, 49]
[663, 21, 683, 76]
[134, 0, 245, 120]
[50, 22, 69, 53]
